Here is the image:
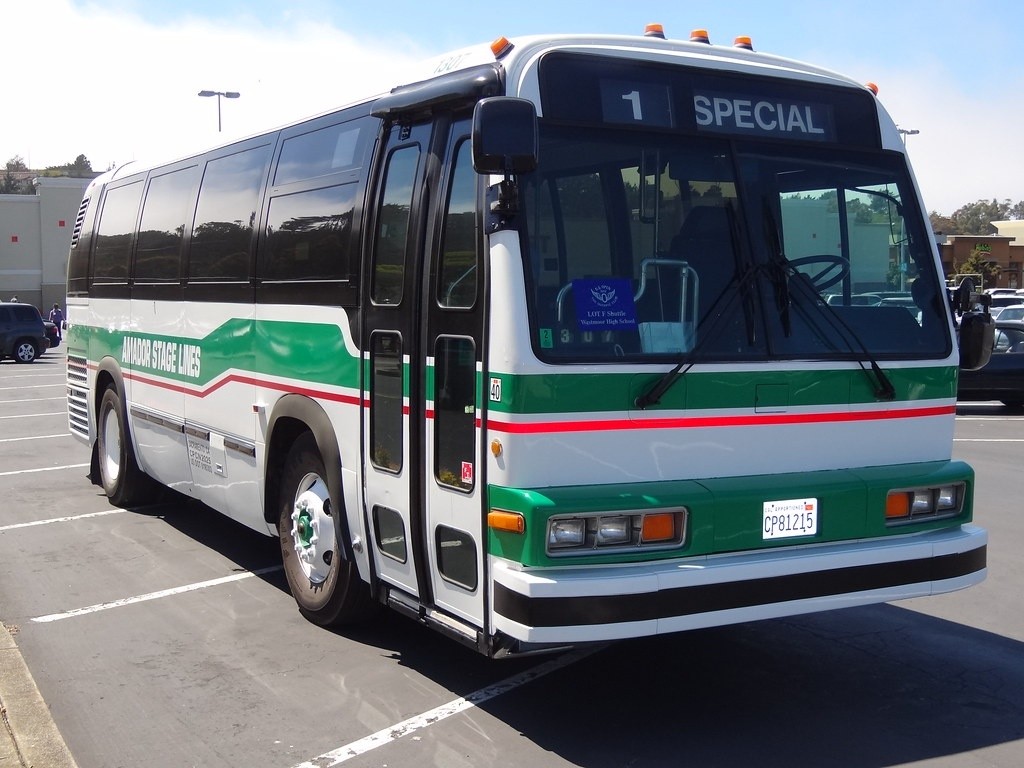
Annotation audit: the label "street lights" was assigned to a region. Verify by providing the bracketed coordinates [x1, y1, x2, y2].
[198, 88, 241, 135]
[895, 126, 920, 293]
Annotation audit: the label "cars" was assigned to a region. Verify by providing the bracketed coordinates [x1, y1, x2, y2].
[44, 322, 61, 348]
[822, 287, 1024, 410]
[0, 303, 51, 363]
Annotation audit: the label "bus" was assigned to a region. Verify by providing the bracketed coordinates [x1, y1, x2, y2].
[65, 20, 990, 659]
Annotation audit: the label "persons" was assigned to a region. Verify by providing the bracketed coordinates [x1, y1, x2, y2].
[49, 303, 65, 340]
[10, 295, 18, 302]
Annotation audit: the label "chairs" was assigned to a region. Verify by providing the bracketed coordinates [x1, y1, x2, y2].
[1005, 341, 1024, 353]
[668, 202, 738, 322]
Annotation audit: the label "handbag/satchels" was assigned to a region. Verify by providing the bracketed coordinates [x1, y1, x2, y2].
[62, 322, 67, 330]
[637, 320, 693, 355]
